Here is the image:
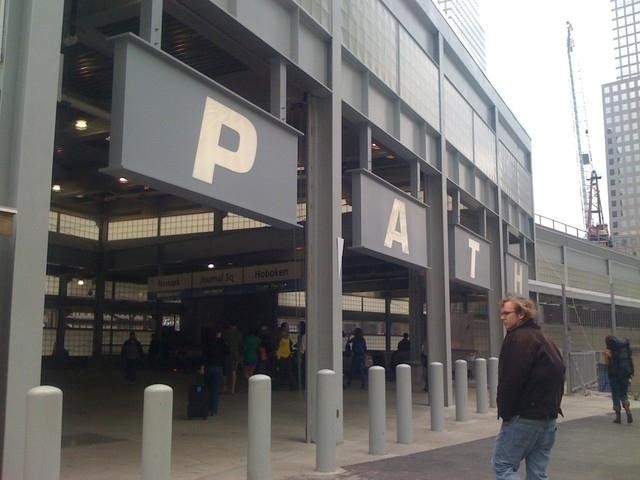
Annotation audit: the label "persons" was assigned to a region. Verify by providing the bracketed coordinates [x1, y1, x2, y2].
[603, 335, 634, 425]
[117, 317, 416, 415]
[491, 293, 566, 480]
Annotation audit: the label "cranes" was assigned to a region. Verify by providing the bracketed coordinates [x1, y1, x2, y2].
[566, 21, 609, 247]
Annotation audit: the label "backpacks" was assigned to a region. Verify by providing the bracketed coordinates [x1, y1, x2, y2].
[607, 335, 633, 384]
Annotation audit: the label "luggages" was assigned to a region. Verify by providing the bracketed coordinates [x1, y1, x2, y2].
[185, 385, 212, 420]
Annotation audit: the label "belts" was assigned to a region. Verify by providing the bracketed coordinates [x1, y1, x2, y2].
[519, 414, 558, 422]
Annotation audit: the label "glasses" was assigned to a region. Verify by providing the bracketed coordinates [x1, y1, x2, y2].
[501, 311, 515, 315]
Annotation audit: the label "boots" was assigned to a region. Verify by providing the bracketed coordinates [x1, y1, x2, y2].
[612, 402, 634, 425]
